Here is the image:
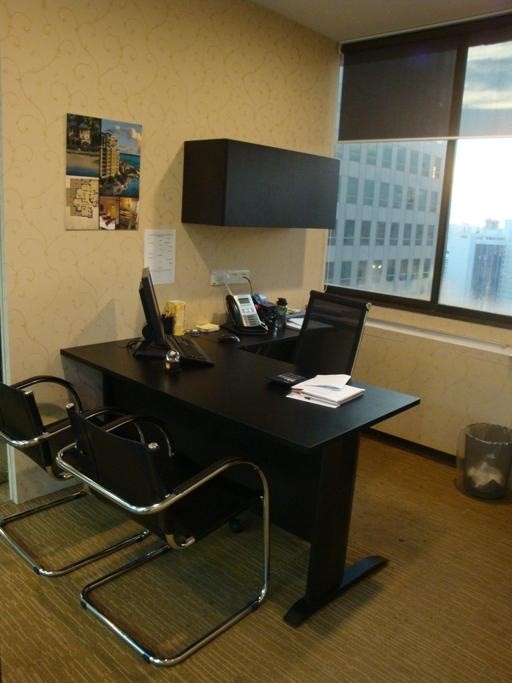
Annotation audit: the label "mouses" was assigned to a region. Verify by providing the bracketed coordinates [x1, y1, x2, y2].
[217, 334, 241, 346]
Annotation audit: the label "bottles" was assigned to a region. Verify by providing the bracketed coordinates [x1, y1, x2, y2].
[274, 297, 288, 327]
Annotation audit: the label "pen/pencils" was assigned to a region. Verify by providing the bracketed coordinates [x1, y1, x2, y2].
[304, 383, 341, 390]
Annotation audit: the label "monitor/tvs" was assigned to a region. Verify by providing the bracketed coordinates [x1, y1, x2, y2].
[133, 266, 172, 360]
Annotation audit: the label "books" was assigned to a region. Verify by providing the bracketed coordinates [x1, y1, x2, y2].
[289, 374, 365, 407]
[284, 316, 336, 331]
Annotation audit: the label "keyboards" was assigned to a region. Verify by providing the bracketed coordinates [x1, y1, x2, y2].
[165, 335, 215, 366]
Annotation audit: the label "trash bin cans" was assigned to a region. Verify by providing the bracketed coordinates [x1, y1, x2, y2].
[461, 423, 511, 506]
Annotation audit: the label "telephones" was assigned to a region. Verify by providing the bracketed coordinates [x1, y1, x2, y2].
[220, 294, 269, 336]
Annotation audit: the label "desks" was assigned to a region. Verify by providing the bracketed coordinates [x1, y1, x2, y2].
[60, 320, 421, 629]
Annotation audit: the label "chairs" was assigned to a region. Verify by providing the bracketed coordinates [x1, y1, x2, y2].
[290, 289, 371, 377]
[0, 375, 270, 667]
